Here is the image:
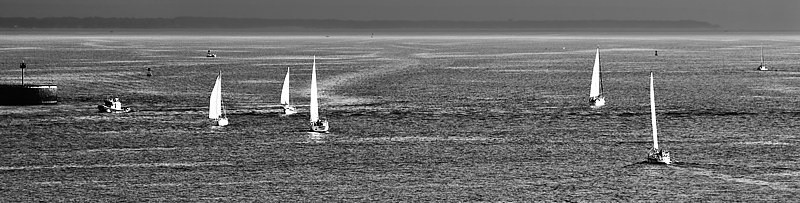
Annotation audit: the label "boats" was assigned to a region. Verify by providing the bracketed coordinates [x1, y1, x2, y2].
[147, 71, 151, 76]
[206, 50, 216, 58]
[98, 97, 131, 114]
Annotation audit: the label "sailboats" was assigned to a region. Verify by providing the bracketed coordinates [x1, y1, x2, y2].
[757, 42, 768, 70]
[589, 44, 606, 108]
[645, 69, 671, 164]
[209, 69, 229, 127]
[309, 54, 329, 133]
[280, 67, 297, 115]
